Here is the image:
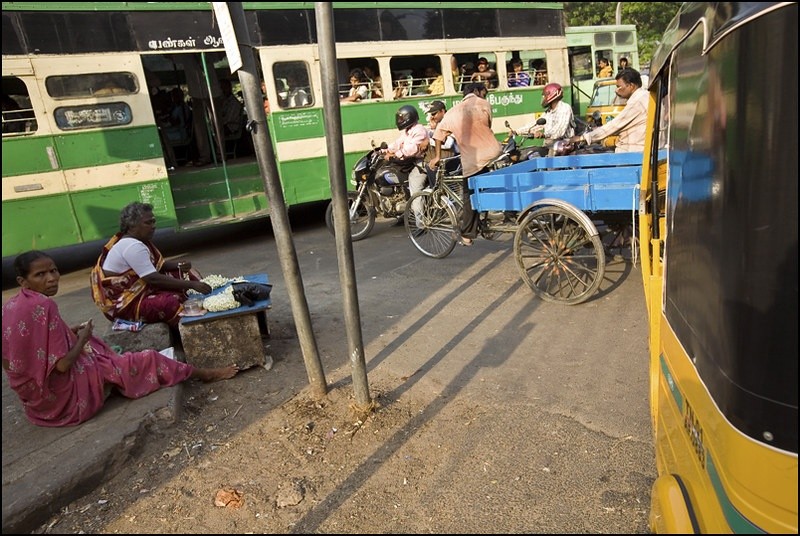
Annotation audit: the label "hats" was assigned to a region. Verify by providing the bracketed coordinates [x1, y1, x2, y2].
[425, 101, 446, 114]
[477, 57, 489, 65]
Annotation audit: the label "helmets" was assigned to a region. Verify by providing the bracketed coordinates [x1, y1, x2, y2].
[396, 105, 419, 131]
[541, 83, 563, 108]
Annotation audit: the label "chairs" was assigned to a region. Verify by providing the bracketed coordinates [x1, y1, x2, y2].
[159, 68, 548, 168]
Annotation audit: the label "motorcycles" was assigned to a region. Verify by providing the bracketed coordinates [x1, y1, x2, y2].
[636, 1, 799, 535]
[585, 76, 628, 149]
[326, 109, 615, 243]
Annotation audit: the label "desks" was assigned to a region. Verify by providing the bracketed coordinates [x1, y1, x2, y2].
[178, 275, 272, 375]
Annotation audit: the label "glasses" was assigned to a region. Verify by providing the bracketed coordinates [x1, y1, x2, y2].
[430, 111, 438, 116]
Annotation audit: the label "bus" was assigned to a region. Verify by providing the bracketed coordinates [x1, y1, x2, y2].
[479, 24, 640, 120]
[0, 1, 573, 259]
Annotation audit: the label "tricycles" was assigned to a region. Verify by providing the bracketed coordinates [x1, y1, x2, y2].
[404, 155, 640, 305]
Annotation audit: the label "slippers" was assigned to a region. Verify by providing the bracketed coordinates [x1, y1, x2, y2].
[453, 227, 474, 246]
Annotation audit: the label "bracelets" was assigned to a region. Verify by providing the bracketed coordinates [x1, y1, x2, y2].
[580, 135, 583, 141]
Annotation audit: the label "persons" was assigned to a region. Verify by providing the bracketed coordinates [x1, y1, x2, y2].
[384, 82, 502, 246]
[261, 72, 309, 115]
[339, 64, 409, 102]
[2, 250, 240, 427]
[515, 83, 575, 171]
[570, 68, 665, 153]
[597, 58, 630, 78]
[156, 52, 243, 169]
[90, 200, 212, 344]
[411, 56, 530, 95]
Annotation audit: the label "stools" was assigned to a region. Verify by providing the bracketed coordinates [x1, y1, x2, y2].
[102, 318, 170, 358]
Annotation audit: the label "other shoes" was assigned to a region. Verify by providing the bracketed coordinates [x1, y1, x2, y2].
[410, 226, 427, 238]
